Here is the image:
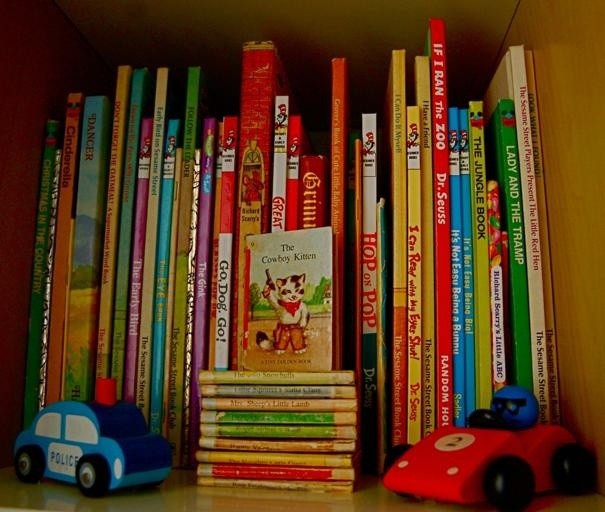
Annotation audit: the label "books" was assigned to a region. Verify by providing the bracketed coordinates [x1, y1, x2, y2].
[22, 17, 563, 496]
